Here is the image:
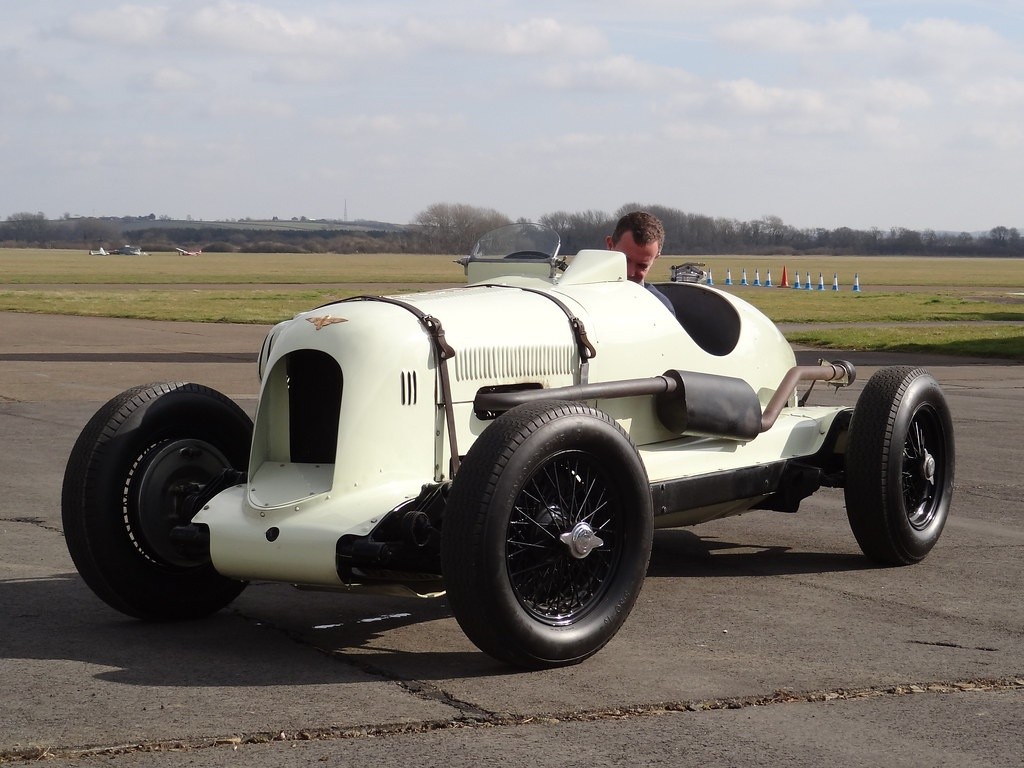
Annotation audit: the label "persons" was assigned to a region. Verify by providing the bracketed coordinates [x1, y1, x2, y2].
[605, 211, 675, 317]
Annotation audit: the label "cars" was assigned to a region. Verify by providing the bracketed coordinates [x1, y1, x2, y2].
[60, 220, 956, 667]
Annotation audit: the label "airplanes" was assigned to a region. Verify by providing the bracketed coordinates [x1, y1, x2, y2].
[175, 246, 202, 256]
[88, 247, 110, 257]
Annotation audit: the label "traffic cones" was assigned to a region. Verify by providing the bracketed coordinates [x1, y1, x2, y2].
[706, 267, 714, 285]
[791, 269, 802, 289]
[804, 270, 813, 290]
[777, 265, 791, 289]
[763, 269, 773, 287]
[751, 268, 761, 286]
[830, 273, 839, 291]
[817, 271, 825, 291]
[723, 266, 734, 285]
[739, 268, 749, 286]
[851, 272, 862, 292]
